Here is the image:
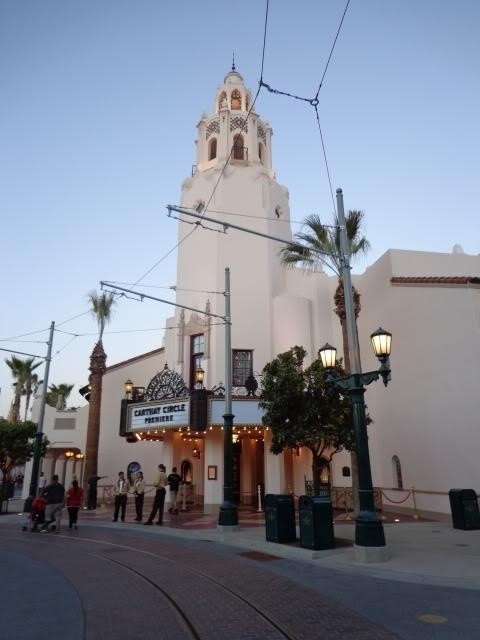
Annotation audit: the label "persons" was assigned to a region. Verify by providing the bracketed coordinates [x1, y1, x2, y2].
[112, 471, 130, 522]
[168, 467, 183, 515]
[133, 472, 146, 521]
[32, 472, 84, 533]
[86, 472, 108, 510]
[14, 472, 25, 489]
[144, 464, 169, 525]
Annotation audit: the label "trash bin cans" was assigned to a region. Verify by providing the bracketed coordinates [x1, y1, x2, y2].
[449, 489, 480, 530]
[264, 494, 296, 543]
[298, 495, 335, 550]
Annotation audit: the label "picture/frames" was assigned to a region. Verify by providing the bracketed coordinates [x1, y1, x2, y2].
[208, 465, 217, 480]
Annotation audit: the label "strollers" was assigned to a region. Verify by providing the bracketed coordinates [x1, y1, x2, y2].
[22, 490, 48, 531]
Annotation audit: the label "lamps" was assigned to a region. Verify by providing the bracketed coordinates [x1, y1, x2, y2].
[193, 445, 200, 459]
[124, 379, 133, 394]
[195, 367, 204, 383]
[64, 449, 73, 456]
[76, 452, 84, 459]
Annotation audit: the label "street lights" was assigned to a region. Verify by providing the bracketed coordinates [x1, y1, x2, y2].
[316, 327, 393, 547]
[63, 450, 83, 483]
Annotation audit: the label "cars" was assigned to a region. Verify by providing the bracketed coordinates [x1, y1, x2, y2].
[0, 469, 16, 500]
